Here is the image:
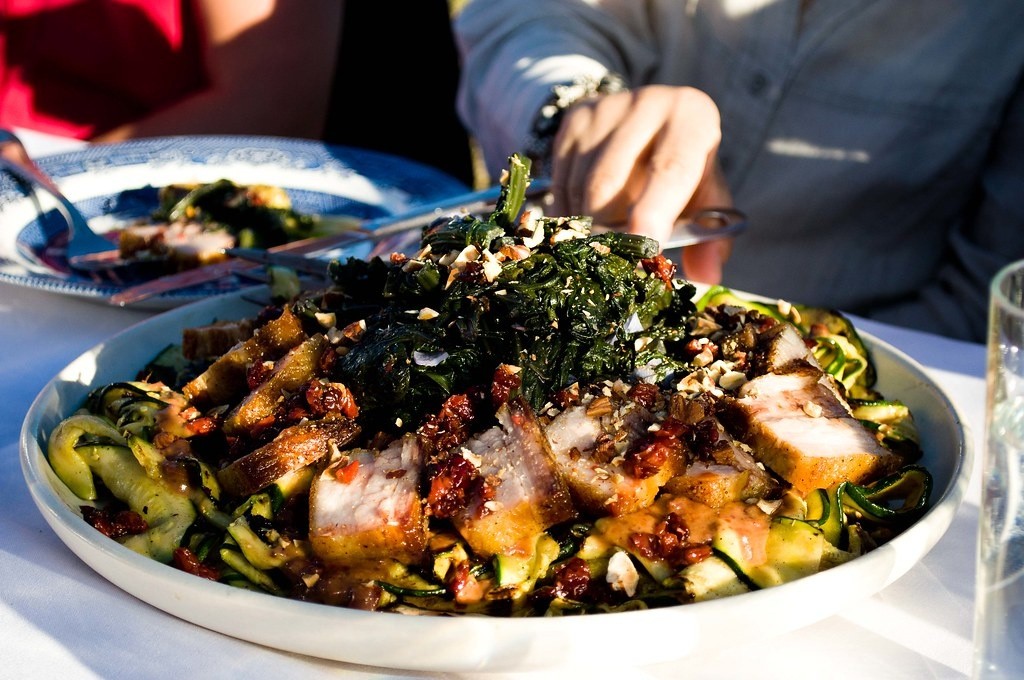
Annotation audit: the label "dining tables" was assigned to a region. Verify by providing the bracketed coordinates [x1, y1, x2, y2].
[2, 128, 1023, 679]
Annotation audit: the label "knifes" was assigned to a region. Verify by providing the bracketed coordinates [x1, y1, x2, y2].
[110, 176, 550, 304]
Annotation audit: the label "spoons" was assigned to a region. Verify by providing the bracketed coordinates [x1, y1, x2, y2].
[1, 126, 134, 271]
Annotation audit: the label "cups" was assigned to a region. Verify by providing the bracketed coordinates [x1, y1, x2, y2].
[969, 259, 1024, 678]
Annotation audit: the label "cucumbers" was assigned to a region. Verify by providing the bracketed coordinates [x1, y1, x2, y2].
[45, 285, 933, 616]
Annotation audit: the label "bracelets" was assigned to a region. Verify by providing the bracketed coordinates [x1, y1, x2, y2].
[524, 73, 626, 178]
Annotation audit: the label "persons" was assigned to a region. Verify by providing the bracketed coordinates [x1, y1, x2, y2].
[0, 1, 341, 148]
[451, 0, 1024, 343]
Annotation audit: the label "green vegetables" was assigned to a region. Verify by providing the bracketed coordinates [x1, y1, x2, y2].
[297, 152, 700, 439]
[157, 179, 328, 251]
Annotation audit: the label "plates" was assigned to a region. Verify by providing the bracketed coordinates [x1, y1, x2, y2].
[0, 134, 474, 309]
[18, 286, 974, 672]
